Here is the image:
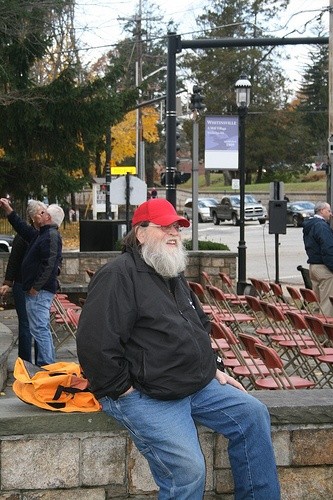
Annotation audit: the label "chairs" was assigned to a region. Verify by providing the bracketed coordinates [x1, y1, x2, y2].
[49, 265, 333, 392]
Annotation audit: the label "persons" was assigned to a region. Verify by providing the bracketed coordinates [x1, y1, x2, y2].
[302, 202, 332, 315]
[0, 197, 64, 368]
[0, 199, 47, 367]
[75, 198, 281, 500]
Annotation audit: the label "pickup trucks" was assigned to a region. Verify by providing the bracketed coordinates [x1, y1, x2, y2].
[210, 195, 266, 227]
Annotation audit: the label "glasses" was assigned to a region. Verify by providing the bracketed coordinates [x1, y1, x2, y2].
[146, 223, 182, 234]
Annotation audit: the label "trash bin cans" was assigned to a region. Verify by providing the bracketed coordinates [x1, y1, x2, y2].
[77, 219, 132, 251]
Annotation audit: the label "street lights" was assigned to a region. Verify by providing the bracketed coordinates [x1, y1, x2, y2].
[232, 72, 253, 295]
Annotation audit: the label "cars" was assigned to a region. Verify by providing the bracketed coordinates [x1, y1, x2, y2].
[285, 199, 318, 228]
[185, 196, 219, 223]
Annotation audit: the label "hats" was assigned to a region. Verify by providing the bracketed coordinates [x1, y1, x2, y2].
[132, 199, 190, 227]
